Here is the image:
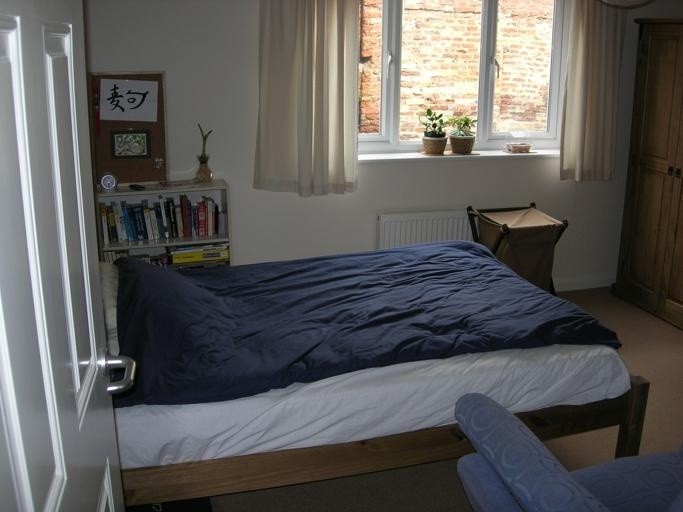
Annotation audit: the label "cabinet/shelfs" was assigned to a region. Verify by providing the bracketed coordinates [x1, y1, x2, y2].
[611, 18, 682, 330]
[95, 179, 234, 273]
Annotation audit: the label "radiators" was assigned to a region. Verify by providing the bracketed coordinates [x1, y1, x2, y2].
[378, 210, 483, 253]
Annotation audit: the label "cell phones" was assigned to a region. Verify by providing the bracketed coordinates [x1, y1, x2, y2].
[129, 184, 145, 190]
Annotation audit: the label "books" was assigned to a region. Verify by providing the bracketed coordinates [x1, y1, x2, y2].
[99, 193, 230, 271]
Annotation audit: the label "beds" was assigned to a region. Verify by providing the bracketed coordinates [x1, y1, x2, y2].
[116, 242, 653, 506]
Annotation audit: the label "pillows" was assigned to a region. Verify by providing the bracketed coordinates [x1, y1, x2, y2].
[92, 258, 126, 360]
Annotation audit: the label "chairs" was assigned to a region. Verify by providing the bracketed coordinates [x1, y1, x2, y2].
[454, 392, 682, 511]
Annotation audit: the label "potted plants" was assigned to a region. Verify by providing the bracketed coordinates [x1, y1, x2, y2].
[421, 108, 477, 155]
[195, 121, 216, 183]
